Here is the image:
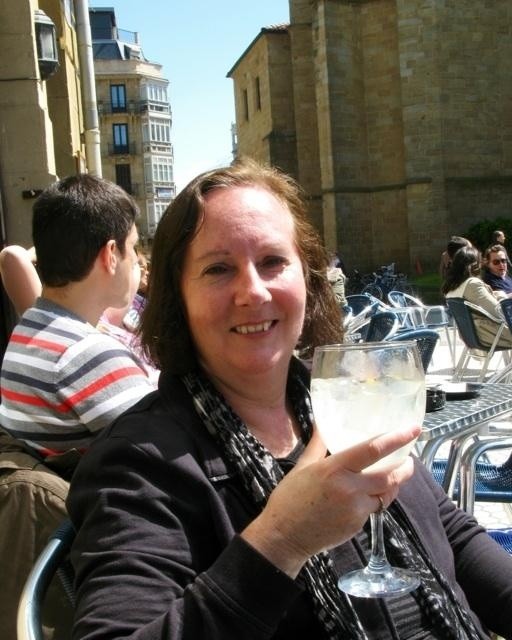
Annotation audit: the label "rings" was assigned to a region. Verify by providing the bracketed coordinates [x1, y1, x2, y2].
[373, 493, 388, 515]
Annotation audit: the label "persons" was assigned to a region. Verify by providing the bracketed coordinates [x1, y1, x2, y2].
[1, 240, 162, 388]
[1, 173, 159, 476]
[436, 220, 512, 351]
[70, 161, 512, 640]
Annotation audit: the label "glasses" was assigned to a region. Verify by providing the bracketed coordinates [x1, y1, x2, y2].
[491, 259, 508, 265]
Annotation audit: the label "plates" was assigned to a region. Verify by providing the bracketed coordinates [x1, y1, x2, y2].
[437, 381, 485, 400]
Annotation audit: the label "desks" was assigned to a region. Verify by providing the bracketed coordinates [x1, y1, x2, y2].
[390, 368, 512, 528]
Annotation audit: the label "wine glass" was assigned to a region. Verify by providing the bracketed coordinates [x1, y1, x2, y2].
[308, 341, 429, 599]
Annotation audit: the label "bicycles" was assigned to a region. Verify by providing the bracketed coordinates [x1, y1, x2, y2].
[346, 264, 418, 305]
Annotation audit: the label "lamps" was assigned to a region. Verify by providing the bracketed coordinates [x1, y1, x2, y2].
[33, 9, 59, 83]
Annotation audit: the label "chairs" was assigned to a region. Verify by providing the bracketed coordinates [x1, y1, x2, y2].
[11, 509, 81, 640]
[2, 440, 83, 640]
[421, 419, 512, 519]
[341, 290, 512, 382]
